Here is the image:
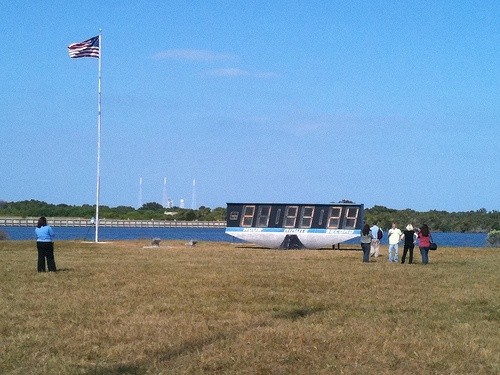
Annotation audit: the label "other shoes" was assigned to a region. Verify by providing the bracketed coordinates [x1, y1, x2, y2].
[408, 262, 412, 264]
[401, 262, 404, 264]
[38, 269, 44, 272]
[49, 269, 56, 272]
[395, 260, 398, 262]
[370, 254, 374, 257]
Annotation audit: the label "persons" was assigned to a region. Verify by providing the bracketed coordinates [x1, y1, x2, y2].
[388, 223, 404, 262]
[361, 224, 372, 262]
[417, 223, 431, 264]
[35, 216, 57, 271]
[370, 222, 384, 257]
[401, 224, 419, 265]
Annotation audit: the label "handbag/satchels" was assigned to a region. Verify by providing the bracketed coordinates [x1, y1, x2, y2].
[378, 229, 382, 239]
[430, 243, 437, 250]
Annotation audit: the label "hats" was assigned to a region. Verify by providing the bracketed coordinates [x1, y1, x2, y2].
[406, 224, 413, 230]
[373, 222, 376, 224]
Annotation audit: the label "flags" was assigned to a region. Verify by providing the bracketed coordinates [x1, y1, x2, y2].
[68, 36, 99, 59]
